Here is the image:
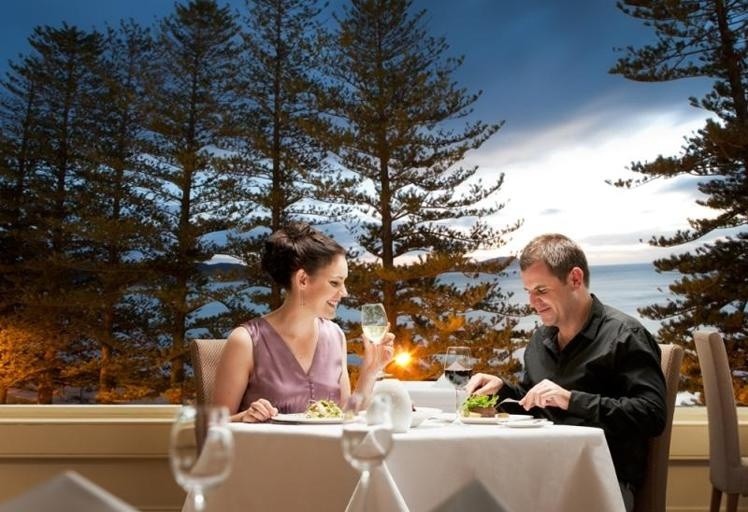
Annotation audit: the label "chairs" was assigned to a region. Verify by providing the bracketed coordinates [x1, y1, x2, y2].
[692, 326, 748, 512]
[189, 338, 228, 458]
[636, 343, 686, 512]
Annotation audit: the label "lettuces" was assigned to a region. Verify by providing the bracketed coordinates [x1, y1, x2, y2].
[463, 392, 499, 417]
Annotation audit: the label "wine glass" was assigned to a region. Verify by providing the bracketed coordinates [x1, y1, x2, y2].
[359, 302, 396, 383]
[440, 344, 474, 428]
[167, 403, 236, 511]
[337, 418, 397, 512]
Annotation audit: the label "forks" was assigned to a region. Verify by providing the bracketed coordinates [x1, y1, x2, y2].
[494, 396, 521, 411]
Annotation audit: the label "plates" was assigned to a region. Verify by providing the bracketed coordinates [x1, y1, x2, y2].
[497, 418, 555, 429]
[270, 411, 364, 426]
[435, 411, 535, 425]
[357, 408, 441, 433]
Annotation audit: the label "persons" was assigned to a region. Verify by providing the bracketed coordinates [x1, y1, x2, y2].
[462, 228, 670, 512]
[205, 219, 397, 424]
